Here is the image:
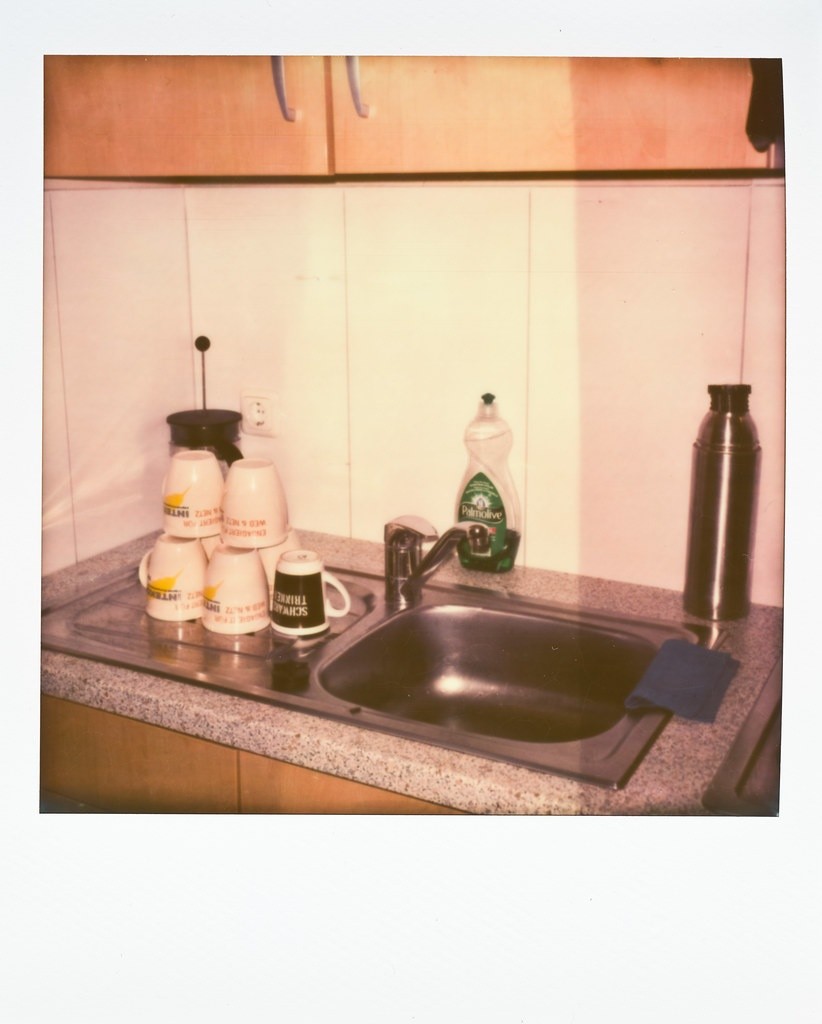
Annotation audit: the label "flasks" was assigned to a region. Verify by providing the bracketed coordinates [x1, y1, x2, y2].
[683, 385, 762, 621]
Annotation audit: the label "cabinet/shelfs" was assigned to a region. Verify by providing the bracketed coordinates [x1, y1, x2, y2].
[45, 55, 784, 182]
[40, 694, 468, 814]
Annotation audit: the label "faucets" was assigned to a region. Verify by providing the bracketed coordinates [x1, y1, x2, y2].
[385, 517, 464, 618]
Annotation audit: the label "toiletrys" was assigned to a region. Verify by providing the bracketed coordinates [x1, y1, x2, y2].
[249, 588, 709, 792]
[454, 394, 522, 574]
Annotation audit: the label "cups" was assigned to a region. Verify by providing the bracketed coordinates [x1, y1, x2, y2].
[269, 550, 351, 635]
[201, 547, 271, 634]
[159, 449, 225, 539]
[259, 531, 302, 585]
[139, 537, 207, 622]
[199, 536, 220, 556]
[219, 458, 288, 548]
